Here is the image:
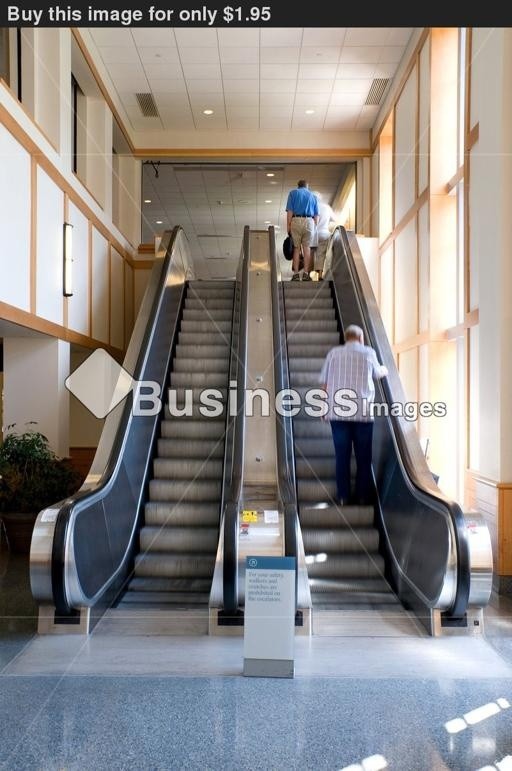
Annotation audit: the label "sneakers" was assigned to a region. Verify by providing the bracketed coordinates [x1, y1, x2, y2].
[291, 274, 324, 281]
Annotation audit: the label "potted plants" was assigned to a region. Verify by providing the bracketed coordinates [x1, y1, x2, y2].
[0, 421, 84, 553]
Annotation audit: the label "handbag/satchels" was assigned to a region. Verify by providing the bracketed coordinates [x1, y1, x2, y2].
[283, 237, 293, 260]
[292, 254, 304, 272]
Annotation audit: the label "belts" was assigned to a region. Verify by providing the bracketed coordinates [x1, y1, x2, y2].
[292, 215, 312, 217]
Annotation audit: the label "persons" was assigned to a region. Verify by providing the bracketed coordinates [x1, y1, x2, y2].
[311, 189, 343, 281]
[285, 180, 319, 281]
[318, 324, 388, 505]
